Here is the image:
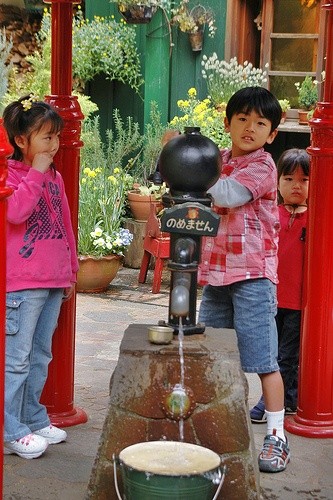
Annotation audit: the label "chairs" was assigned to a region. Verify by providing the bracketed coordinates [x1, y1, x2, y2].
[138, 202, 171, 293]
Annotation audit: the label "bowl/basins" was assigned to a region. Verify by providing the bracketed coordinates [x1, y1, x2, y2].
[147, 325, 175, 346]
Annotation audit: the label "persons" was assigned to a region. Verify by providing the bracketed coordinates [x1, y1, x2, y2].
[162, 87, 291, 472]
[3, 92, 79, 460]
[250, 148, 310, 424]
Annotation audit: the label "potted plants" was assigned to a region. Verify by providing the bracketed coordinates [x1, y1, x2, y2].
[278, 98, 292, 125]
[295, 74, 317, 125]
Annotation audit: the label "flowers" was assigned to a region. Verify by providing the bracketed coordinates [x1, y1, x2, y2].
[171, 0, 218, 39]
[170, 52, 271, 142]
[108, 0, 176, 13]
[76, 166, 135, 258]
[21, 99, 33, 112]
[3, 3, 142, 99]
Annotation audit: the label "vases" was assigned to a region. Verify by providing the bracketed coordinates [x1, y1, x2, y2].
[116, 3, 158, 24]
[188, 31, 203, 51]
[76, 254, 123, 293]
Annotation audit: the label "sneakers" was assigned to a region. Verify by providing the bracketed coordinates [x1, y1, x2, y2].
[257, 432, 291, 472]
[250, 393, 268, 422]
[33, 424, 67, 444]
[3, 432, 48, 460]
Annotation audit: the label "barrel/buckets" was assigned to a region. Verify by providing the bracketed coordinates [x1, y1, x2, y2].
[109, 440, 227, 496]
